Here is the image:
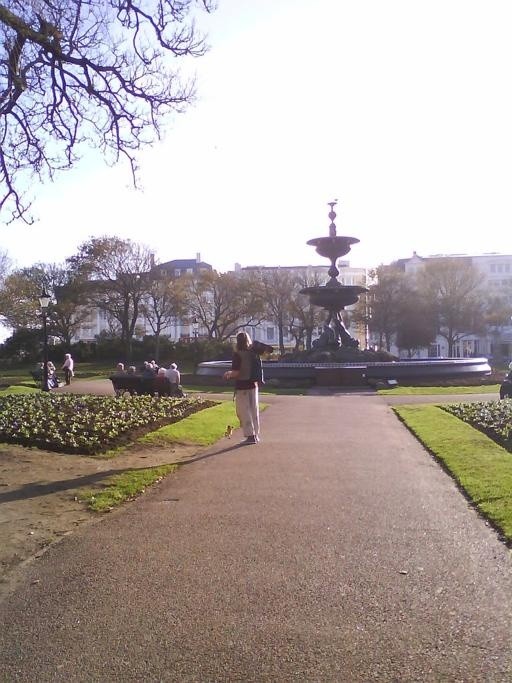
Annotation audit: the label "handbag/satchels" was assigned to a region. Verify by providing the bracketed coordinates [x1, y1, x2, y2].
[250, 359, 262, 382]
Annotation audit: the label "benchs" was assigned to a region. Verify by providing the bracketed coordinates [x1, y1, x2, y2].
[30, 370, 44, 384]
[110, 374, 168, 397]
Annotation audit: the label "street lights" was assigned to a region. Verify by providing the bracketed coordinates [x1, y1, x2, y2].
[192, 317, 201, 375]
[39, 285, 52, 392]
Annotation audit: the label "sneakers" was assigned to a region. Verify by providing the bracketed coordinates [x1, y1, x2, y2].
[240, 435, 260, 444]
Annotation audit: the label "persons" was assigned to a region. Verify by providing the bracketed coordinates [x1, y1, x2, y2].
[224, 331, 259, 443]
[40, 353, 73, 390]
[111, 360, 181, 395]
